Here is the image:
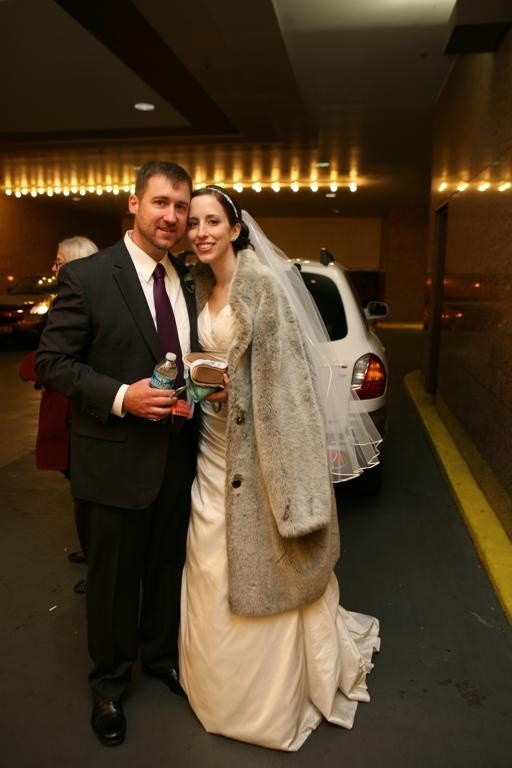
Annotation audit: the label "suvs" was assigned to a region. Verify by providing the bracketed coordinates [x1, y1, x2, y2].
[175, 246, 391, 496]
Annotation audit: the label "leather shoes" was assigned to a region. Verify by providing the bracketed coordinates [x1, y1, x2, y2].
[69, 551, 86, 564]
[74, 580, 87, 592]
[182, 353, 228, 388]
[90, 692, 126, 747]
[145, 664, 184, 695]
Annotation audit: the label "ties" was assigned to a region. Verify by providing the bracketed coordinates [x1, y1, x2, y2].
[154, 262, 188, 432]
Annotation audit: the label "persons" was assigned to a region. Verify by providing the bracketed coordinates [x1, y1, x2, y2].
[18, 237, 101, 595]
[174, 183, 384, 753]
[38, 158, 230, 745]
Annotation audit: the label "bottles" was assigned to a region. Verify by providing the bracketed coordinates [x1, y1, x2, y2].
[144, 350, 179, 422]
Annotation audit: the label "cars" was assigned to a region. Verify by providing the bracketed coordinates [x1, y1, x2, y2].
[423, 293, 499, 335]
[1, 273, 57, 341]
